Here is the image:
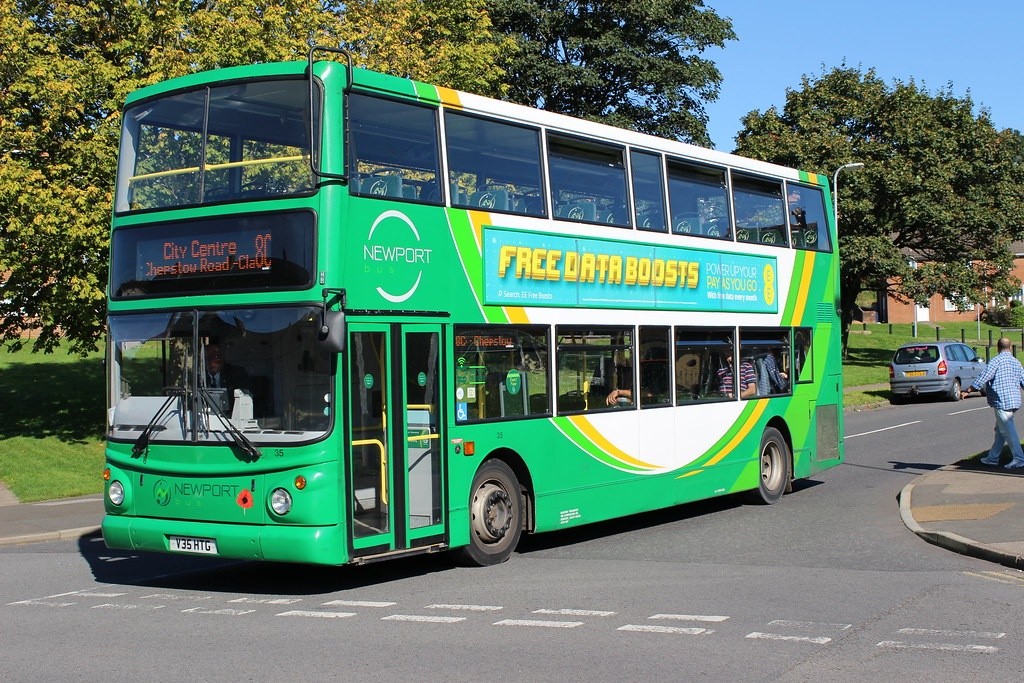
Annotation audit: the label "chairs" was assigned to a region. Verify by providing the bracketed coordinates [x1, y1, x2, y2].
[745, 354, 769, 396]
[205, 167, 826, 249]
[676, 354, 700, 391]
[529, 385, 611, 414]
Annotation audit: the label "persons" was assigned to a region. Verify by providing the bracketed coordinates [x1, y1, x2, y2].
[716, 345, 789, 399]
[606, 354, 654, 405]
[961, 337, 1024, 468]
[189, 345, 253, 417]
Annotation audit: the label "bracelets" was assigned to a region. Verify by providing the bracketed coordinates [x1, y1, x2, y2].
[615, 389, 619, 396]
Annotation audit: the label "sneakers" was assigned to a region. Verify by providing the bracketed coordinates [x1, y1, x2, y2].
[1004, 461, 1024, 469]
[981, 458, 999, 465]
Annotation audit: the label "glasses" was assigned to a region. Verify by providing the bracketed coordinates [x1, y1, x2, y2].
[206, 354, 220, 360]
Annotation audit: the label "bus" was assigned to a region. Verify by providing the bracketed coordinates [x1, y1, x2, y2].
[101, 45, 845, 567]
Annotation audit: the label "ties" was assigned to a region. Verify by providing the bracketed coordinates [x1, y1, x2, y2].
[212, 376, 217, 388]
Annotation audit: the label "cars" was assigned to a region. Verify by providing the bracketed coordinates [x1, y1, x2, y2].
[889, 344, 986, 401]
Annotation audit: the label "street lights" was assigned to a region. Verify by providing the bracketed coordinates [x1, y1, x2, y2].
[835, 163, 864, 237]
